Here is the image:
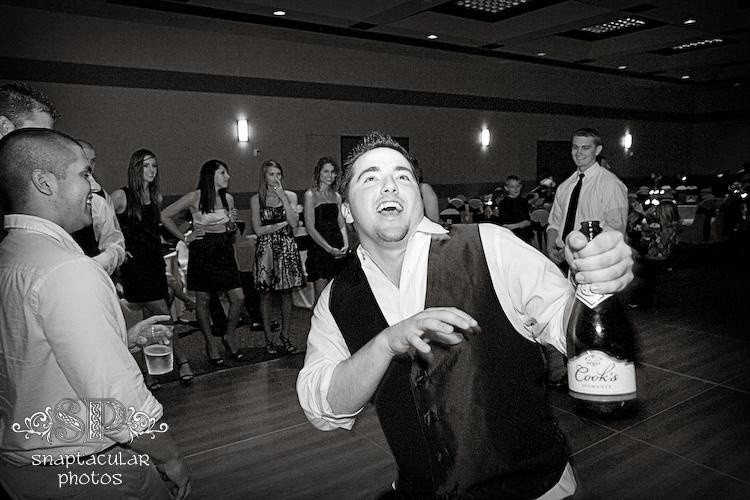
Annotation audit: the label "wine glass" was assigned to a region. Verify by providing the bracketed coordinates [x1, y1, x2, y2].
[224, 206, 238, 231]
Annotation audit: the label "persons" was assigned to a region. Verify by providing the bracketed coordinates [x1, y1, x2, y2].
[600, 156, 611, 168]
[0, 81, 303, 500]
[497, 175, 533, 246]
[304, 157, 349, 307]
[545, 127, 629, 387]
[408, 158, 439, 224]
[298, 137, 635, 500]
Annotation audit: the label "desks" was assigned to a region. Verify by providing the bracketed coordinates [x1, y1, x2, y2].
[676, 205, 703, 221]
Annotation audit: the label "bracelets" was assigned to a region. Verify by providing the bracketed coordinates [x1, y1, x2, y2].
[330, 247, 334, 254]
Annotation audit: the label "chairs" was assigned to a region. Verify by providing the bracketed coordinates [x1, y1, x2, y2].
[711, 195, 744, 241]
[172, 219, 319, 312]
[440, 188, 557, 257]
[677, 199, 716, 244]
[734, 194, 750, 238]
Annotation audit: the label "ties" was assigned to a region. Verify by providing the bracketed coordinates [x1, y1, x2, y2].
[562, 174, 584, 244]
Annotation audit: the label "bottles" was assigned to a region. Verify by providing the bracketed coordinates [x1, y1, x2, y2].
[564, 219, 642, 422]
[460, 203, 473, 224]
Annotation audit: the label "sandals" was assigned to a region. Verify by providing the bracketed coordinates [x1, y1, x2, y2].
[177, 334, 296, 380]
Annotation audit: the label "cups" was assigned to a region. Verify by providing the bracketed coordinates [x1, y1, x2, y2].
[140, 324, 177, 377]
